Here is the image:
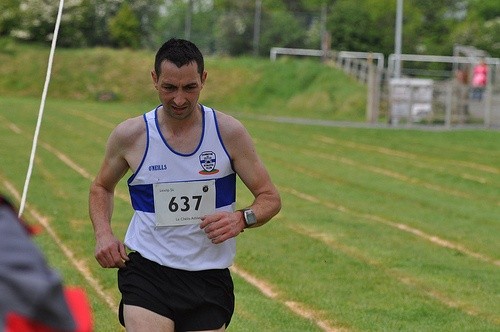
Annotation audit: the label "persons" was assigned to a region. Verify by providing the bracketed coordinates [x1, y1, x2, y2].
[88, 35, 282, 332]
[470, 53, 489, 102]
[0, 192, 79, 332]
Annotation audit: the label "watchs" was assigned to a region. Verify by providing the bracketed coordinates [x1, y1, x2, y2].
[238, 208, 258, 232]
